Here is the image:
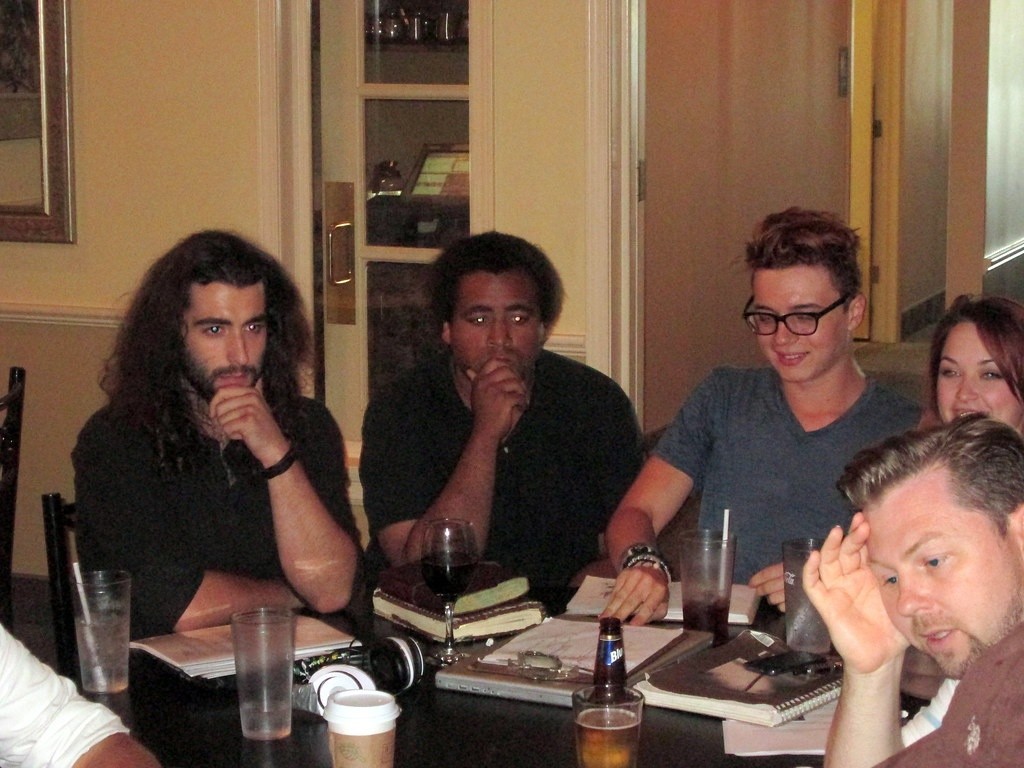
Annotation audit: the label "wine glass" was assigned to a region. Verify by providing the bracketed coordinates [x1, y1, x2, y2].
[421, 519, 476, 666]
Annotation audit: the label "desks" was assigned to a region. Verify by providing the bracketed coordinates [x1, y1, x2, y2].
[80, 586, 930, 768]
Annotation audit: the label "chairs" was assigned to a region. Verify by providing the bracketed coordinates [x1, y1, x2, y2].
[0, 366, 26, 633]
[38, 491, 79, 678]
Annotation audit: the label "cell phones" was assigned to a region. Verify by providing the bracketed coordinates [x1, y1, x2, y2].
[743, 649, 827, 675]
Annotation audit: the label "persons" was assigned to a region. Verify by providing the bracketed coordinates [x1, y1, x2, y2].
[0, 624, 163, 768]
[802, 414, 1024, 767]
[71, 229, 361, 626]
[356, 234, 641, 567]
[902, 294, 1024, 703]
[604, 209, 932, 625]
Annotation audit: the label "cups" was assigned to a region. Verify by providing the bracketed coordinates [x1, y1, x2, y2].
[782, 538, 832, 654]
[321, 691, 399, 768]
[678, 529, 735, 643]
[572, 686, 644, 768]
[366, 8, 461, 40]
[231, 606, 293, 739]
[73, 568, 129, 693]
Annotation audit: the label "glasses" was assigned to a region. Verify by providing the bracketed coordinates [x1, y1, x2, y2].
[741, 291, 851, 336]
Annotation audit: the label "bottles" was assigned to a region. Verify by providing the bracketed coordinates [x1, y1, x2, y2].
[581, 615, 637, 712]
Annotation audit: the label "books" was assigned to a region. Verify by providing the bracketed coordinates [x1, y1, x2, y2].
[564, 575, 683, 621]
[634, 629, 843, 729]
[436, 615, 713, 707]
[370, 558, 543, 645]
[129, 615, 362, 680]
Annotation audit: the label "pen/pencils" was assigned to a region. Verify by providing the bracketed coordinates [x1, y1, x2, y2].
[793, 664, 842, 676]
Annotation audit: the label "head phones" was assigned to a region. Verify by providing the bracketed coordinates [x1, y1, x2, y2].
[290, 635, 424, 717]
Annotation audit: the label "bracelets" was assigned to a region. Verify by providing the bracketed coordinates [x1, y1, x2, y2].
[262, 440, 299, 479]
[620, 543, 671, 586]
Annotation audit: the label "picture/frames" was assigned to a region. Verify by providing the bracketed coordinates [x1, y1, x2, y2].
[0, 0, 78, 246]
